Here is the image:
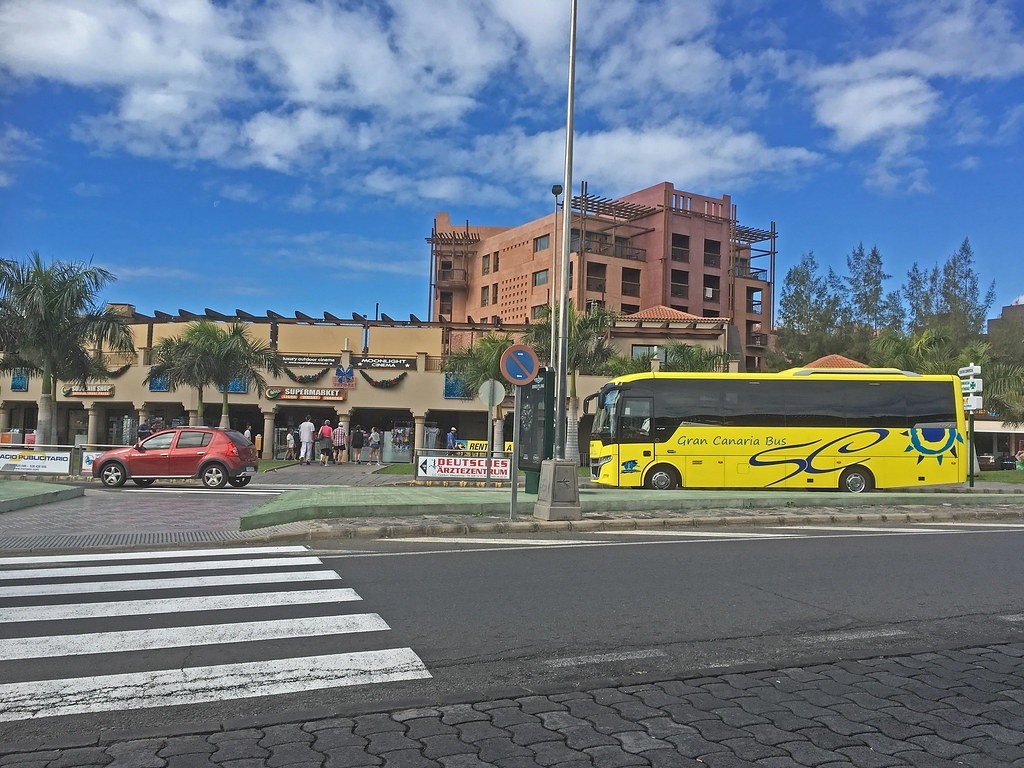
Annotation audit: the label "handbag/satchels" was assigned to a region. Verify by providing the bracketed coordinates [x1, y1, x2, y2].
[319, 426, 323, 440]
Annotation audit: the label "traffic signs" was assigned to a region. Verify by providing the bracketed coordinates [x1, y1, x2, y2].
[963, 396, 984, 411]
[961, 379, 983, 393]
[957, 366, 982, 377]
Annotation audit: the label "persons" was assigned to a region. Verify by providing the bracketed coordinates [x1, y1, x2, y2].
[351, 425, 364, 465]
[284, 428, 302, 461]
[447, 427, 459, 456]
[629, 409, 650, 436]
[366, 427, 380, 465]
[333, 422, 348, 465]
[138, 419, 161, 441]
[243, 425, 252, 442]
[318, 420, 333, 466]
[299, 415, 315, 465]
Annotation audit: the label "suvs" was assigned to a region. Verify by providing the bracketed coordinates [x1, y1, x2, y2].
[91, 424, 259, 489]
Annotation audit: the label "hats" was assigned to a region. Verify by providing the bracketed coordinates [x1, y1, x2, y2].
[451, 427, 456, 430]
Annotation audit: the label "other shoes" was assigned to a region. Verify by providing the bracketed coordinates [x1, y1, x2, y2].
[355, 460, 361, 465]
[325, 464, 330, 467]
[376, 463, 380, 466]
[320, 460, 323, 466]
[300, 457, 303, 465]
[367, 462, 371, 466]
[307, 461, 310, 465]
[338, 462, 342, 465]
[333, 459, 335, 464]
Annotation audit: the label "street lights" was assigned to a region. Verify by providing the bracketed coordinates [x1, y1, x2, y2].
[550, 183, 565, 370]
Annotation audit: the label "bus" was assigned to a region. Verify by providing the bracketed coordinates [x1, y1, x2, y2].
[582, 366, 969, 492]
